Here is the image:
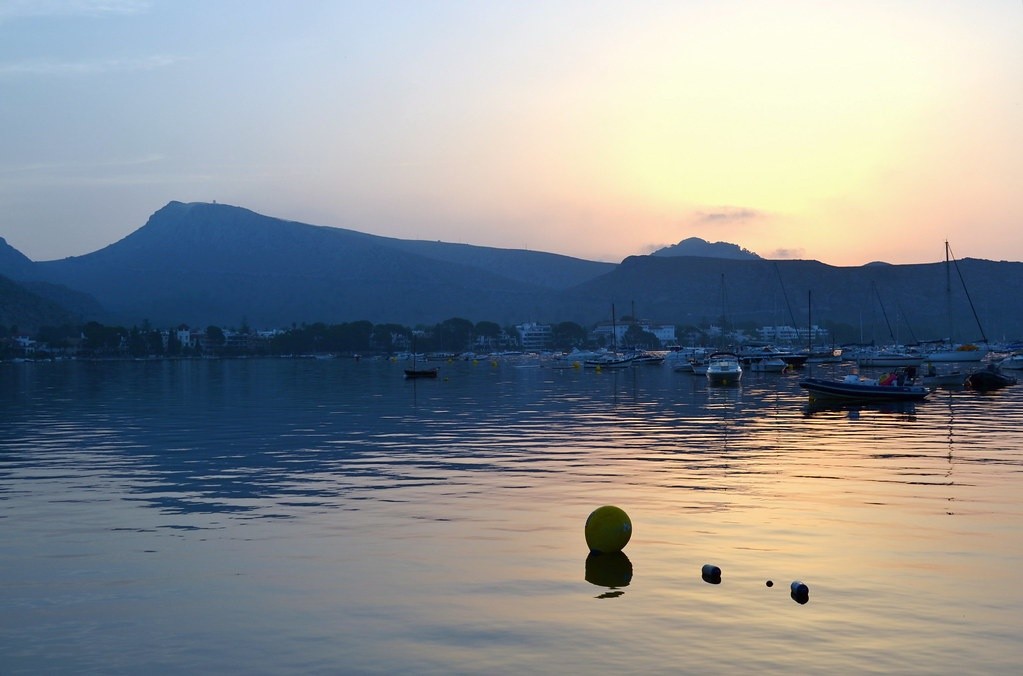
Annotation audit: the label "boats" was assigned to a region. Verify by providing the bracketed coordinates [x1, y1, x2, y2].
[373, 299, 665, 369]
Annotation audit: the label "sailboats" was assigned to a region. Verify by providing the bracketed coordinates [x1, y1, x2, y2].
[672, 239, 1023, 406]
[402, 333, 437, 377]
